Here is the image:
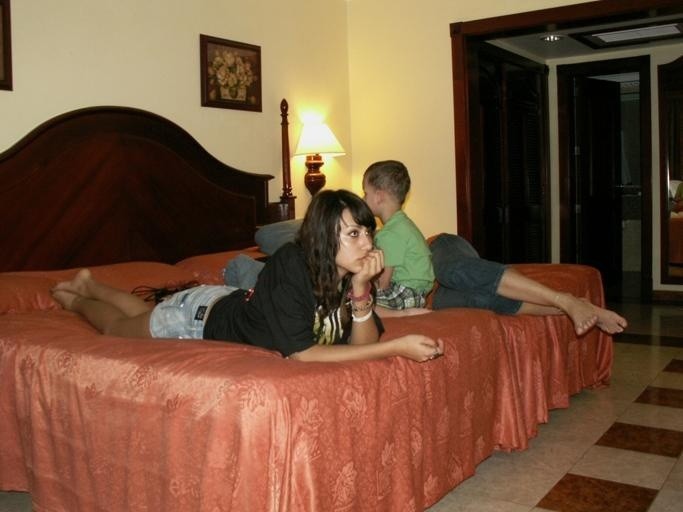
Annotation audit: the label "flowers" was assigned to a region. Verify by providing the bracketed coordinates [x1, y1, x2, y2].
[210, 46, 258, 90]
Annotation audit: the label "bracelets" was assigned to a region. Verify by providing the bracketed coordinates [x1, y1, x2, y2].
[347, 282, 371, 302]
[351, 308, 373, 323]
[349, 295, 374, 312]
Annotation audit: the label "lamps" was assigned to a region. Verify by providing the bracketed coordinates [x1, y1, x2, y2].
[290, 124, 348, 199]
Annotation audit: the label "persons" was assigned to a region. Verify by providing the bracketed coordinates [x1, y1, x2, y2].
[423, 233, 628, 336]
[51, 190, 444, 362]
[362, 161, 435, 310]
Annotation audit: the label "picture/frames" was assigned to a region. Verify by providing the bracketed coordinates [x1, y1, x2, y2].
[200, 33, 263, 113]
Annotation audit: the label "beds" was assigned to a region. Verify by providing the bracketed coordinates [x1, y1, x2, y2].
[0, 105, 615, 511]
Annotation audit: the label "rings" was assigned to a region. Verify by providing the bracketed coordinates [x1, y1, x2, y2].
[433, 348, 439, 359]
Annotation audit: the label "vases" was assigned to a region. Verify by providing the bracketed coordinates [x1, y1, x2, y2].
[220, 86, 248, 103]
